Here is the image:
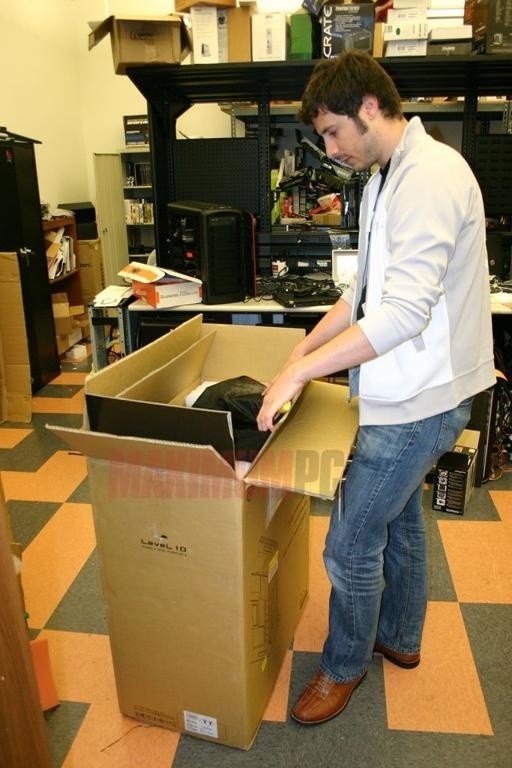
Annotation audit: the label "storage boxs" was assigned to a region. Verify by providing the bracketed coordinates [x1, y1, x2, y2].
[51, 238, 104, 355]
[46, 313, 359, 752]
[87, 15, 184, 75]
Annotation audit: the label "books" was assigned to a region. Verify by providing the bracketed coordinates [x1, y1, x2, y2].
[125, 198, 154, 225]
[125, 119, 149, 145]
[124, 162, 151, 185]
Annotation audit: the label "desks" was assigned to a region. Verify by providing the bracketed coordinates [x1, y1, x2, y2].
[128, 275, 512, 375]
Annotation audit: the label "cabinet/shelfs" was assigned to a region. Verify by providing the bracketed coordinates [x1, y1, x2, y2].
[43, 216, 91, 359]
[230, 102, 512, 245]
[93, 144, 158, 289]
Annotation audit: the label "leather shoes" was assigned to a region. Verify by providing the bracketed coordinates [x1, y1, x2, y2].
[373, 639, 421, 669]
[289, 667, 368, 728]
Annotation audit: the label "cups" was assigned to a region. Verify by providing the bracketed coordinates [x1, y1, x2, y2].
[271, 261, 289, 279]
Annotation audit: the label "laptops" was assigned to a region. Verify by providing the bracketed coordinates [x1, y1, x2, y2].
[272, 292, 342, 308]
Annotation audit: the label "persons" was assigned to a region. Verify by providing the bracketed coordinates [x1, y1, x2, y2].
[256, 49, 497, 725]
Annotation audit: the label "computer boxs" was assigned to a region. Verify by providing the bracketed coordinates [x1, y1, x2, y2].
[164, 200, 248, 305]
